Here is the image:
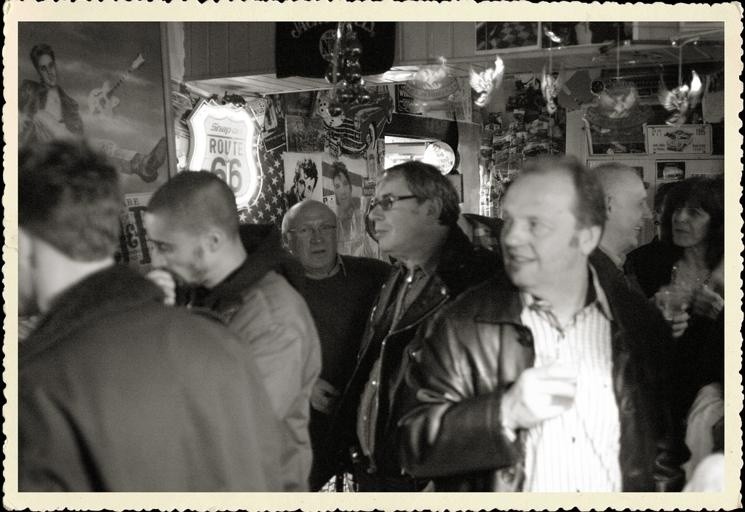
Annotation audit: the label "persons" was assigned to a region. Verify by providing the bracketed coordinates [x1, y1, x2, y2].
[17, 142, 307, 494]
[276, 197, 393, 499]
[145, 171, 323, 478]
[387, 151, 693, 495]
[337, 160, 482, 488]
[624, 176, 723, 387]
[18, 44, 167, 182]
[587, 161, 661, 264]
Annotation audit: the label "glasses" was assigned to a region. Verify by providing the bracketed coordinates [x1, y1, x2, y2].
[369, 194, 416, 210]
[288, 225, 336, 234]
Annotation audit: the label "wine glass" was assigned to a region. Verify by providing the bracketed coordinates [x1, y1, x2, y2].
[319, 30, 363, 101]
[548, 375, 576, 447]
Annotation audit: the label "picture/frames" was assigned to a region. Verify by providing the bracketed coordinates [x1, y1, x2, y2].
[21, 23, 179, 279]
[475, 21, 617, 56]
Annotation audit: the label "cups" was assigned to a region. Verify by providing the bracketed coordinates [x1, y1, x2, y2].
[656, 288, 694, 315]
